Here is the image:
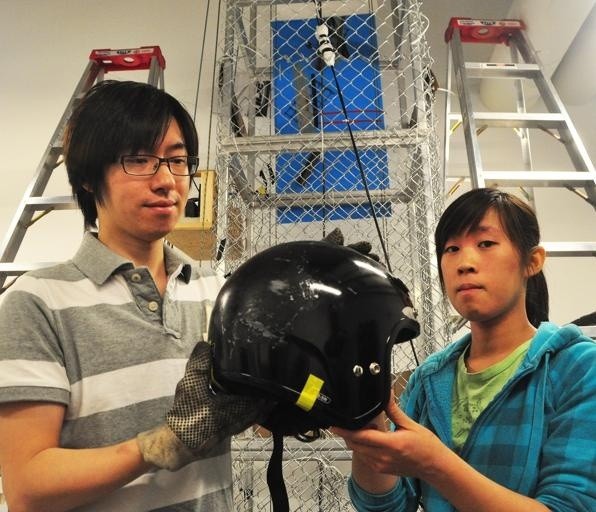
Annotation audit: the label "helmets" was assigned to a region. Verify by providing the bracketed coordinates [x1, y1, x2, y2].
[207, 241, 420, 428]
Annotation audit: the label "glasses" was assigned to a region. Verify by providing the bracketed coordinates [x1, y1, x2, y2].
[121, 153, 200, 177]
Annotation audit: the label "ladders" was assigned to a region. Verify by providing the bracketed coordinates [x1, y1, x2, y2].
[445, 18, 595, 339]
[0, 45, 166, 314]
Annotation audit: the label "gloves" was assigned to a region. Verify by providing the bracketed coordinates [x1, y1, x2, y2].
[326, 227, 381, 263]
[137, 343, 265, 471]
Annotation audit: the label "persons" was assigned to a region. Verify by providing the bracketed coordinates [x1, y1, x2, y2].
[0, 79, 388, 512]
[326, 188, 596, 512]
